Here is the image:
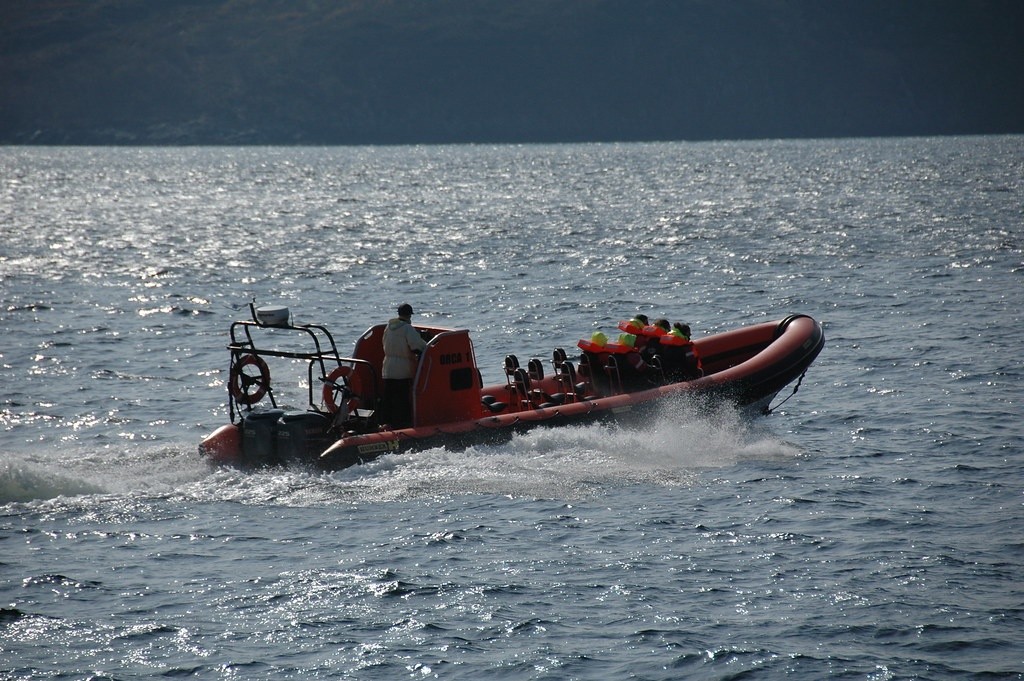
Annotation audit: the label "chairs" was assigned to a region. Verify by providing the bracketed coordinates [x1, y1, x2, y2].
[474, 368, 508, 414]
[511, 368, 556, 410]
[501, 354, 526, 398]
[559, 360, 598, 403]
[549, 348, 579, 401]
[603, 355, 639, 395]
[527, 358, 566, 404]
[576, 351, 607, 394]
[647, 354, 680, 386]
[482, 394, 496, 404]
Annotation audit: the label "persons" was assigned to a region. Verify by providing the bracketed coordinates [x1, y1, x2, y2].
[382, 303, 428, 427]
[577, 315, 703, 384]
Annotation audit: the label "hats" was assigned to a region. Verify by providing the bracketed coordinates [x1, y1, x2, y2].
[396, 304, 415, 315]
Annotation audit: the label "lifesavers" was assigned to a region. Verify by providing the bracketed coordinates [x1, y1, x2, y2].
[322, 366, 362, 417]
[228, 355, 271, 404]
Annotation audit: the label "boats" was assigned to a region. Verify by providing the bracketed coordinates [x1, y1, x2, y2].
[195, 298, 826, 481]
